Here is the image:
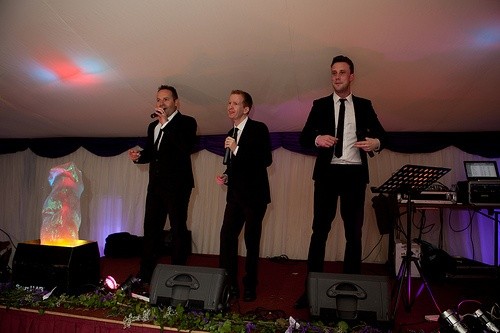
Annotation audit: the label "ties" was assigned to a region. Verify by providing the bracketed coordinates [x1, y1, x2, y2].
[231, 128, 239, 162]
[154, 129, 162, 148]
[334, 99, 347, 158]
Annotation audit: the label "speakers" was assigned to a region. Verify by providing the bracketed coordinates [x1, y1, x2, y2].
[149, 263, 232, 315]
[306, 271, 390, 327]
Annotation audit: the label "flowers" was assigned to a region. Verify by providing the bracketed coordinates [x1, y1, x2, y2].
[0, 280, 394, 333]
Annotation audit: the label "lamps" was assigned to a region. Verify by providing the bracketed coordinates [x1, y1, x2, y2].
[98, 275, 118, 296]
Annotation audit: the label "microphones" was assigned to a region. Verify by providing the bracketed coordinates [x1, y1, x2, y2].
[151, 108, 166, 118]
[355, 129, 374, 158]
[223, 135, 233, 164]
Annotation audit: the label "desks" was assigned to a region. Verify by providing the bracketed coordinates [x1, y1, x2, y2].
[388, 201, 500, 282]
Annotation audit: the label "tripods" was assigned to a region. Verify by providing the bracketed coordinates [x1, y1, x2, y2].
[369, 164, 452, 325]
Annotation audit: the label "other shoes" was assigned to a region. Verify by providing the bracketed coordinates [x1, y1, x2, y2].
[243, 287, 256, 301]
[135, 272, 152, 283]
[294, 292, 309, 309]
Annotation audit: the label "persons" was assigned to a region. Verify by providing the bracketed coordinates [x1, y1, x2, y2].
[127, 85, 199, 291]
[290, 55, 388, 311]
[216, 90, 273, 301]
